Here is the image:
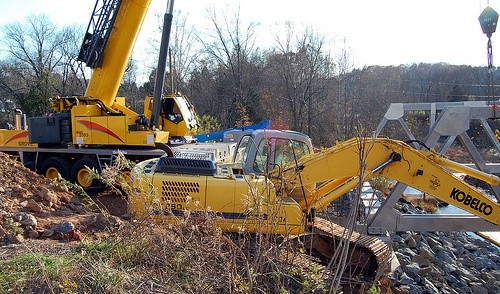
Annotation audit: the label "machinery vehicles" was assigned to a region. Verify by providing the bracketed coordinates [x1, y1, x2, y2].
[122, 126, 500, 294]
[0, 0, 206, 201]
